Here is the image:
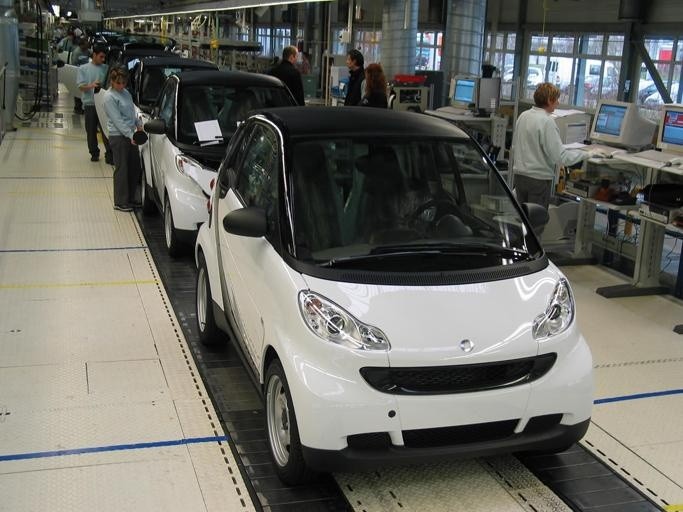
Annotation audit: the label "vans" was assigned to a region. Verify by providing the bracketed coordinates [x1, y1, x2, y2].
[548, 56, 618, 92]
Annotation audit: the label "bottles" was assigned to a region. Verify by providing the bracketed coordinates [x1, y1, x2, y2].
[636, 189, 645, 208]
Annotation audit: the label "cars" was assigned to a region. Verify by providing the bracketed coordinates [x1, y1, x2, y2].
[580, 74, 679, 110]
[504, 67, 548, 88]
[192, 102, 594, 484]
[135, 70, 299, 260]
[54, 28, 175, 133]
[130, 55, 220, 170]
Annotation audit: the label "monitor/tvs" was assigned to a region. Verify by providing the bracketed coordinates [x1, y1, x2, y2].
[590, 99, 657, 154]
[656, 104, 683, 156]
[451, 74, 479, 110]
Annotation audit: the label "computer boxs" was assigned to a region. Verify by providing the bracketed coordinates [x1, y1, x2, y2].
[331, 66, 351, 86]
[565, 178, 600, 198]
[639, 201, 682, 224]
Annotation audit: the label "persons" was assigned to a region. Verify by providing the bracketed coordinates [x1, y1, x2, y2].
[357, 64, 387, 108]
[58, 25, 93, 66]
[261, 45, 304, 106]
[103, 68, 144, 211]
[511, 83, 609, 242]
[76, 47, 110, 162]
[344, 49, 365, 105]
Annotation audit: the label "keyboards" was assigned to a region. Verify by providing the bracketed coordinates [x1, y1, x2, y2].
[436, 104, 467, 115]
[581, 145, 627, 156]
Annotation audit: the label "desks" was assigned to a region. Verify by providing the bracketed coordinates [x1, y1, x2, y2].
[484, 140, 683, 336]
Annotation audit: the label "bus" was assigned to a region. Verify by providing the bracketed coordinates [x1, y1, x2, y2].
[359, 33, 432, 70]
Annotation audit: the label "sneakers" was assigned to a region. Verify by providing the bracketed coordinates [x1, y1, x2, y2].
[91, 155, 100, 161]
[113, 204, 133, 211]
[128, 200, 142, 207]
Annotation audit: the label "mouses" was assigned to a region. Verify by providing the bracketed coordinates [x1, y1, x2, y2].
[600, 153, 613, 159]
